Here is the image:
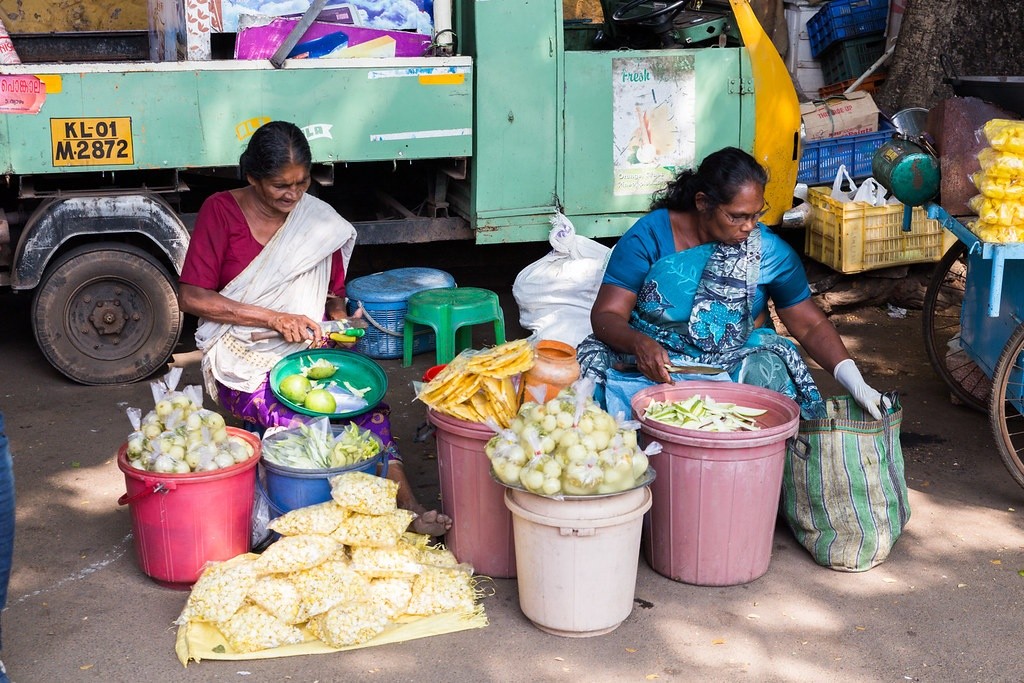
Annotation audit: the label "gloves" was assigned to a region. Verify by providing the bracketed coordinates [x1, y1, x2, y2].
[834, 359, 893, 421]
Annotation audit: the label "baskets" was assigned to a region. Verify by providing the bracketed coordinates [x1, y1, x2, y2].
[345, 267, 456, 359]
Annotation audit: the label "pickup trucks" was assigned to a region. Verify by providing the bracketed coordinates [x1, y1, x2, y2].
[0, 0, 803, 388]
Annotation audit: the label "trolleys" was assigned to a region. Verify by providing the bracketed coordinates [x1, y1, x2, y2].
[901, 202, 1024, 486]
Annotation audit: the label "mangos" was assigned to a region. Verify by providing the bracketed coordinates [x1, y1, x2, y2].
[329, 333, 356, 343]
[279, 375, 336, 413]
[307, 358, 336, 378]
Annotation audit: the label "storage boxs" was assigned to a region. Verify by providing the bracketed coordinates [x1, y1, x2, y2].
[784, 9, 826, 91]
[818, 73, 887, 98]
[805, 187, 945, 275]
[805, 0, 889, 58]
[797, 119, 897, 184]
[800, 90, 882, 141]
[821, 33, 889, 85]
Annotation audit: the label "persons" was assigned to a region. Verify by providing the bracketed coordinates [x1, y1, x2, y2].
[0, 412, 15, 683]
[178, 120, 453, 546]
[576, 146, 891, 424]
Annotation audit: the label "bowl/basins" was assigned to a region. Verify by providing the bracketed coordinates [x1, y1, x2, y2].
[269, 348, 389, 418]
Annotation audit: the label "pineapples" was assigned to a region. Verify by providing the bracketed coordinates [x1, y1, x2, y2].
[415, 338, 535, 429]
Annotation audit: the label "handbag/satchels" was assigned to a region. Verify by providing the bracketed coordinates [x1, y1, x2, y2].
[782, 390, 911, 572]
[830, 164, 888, 206]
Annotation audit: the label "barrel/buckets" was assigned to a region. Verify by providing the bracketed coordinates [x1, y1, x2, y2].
[426, 405, 517, 578]
[117, 426, 262, 589]
[256, 424, 388, 540]
[503, 487, 653, 638]
[630, 381, 800, 586]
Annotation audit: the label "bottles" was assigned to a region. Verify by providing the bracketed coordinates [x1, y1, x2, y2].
[515, 339, 580, 413]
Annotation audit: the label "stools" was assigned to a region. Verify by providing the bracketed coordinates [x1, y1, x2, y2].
[404, 287, 505, 368]
